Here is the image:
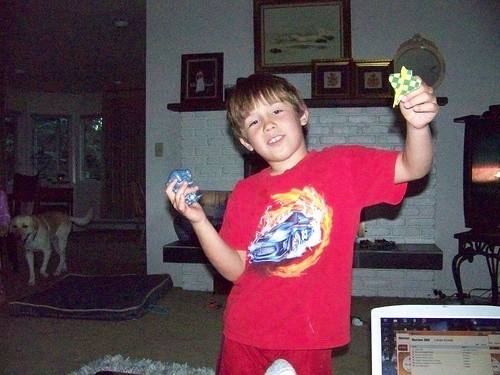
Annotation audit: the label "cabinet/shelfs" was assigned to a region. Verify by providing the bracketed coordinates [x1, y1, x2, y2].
[163, 97, 448, 271]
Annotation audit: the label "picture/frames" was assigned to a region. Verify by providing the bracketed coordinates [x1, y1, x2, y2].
[310, 58, 353, 96]
[252, 0, 352, 74]
[180, 52, 226, 102]
[353, 56, 394, 94]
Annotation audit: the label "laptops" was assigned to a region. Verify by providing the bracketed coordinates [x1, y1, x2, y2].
[370, 305, 500, 375]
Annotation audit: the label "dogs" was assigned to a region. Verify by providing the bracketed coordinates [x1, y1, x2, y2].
[8, 205, 96, 286]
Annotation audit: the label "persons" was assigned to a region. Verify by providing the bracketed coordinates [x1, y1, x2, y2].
[165, 72, 439, 375]
[0, 190, 11, 237]
[196, 72, 205, 97]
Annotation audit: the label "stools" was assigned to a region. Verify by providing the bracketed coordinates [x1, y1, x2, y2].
[452, 232, 500, 305]
